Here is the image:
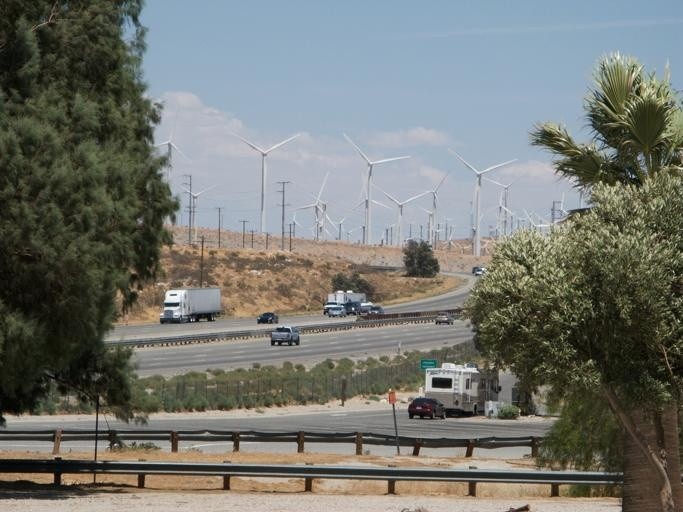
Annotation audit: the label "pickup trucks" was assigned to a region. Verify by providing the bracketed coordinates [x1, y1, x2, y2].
[271, 326, 300, 345]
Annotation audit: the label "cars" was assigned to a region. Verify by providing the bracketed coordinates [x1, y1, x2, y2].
[324, 301, 384, 318]
[435, 312, 454, 325]
[472, 266, 485, 276]
[408, 398, 446, 419]
[257, 312, 278, 324]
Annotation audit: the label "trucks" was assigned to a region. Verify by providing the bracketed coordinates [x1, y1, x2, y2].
[160, 288, 221, 324]
[328, 290, 366, 304]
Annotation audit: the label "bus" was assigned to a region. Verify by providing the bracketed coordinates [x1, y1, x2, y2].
[425, 363, 502, 416]
